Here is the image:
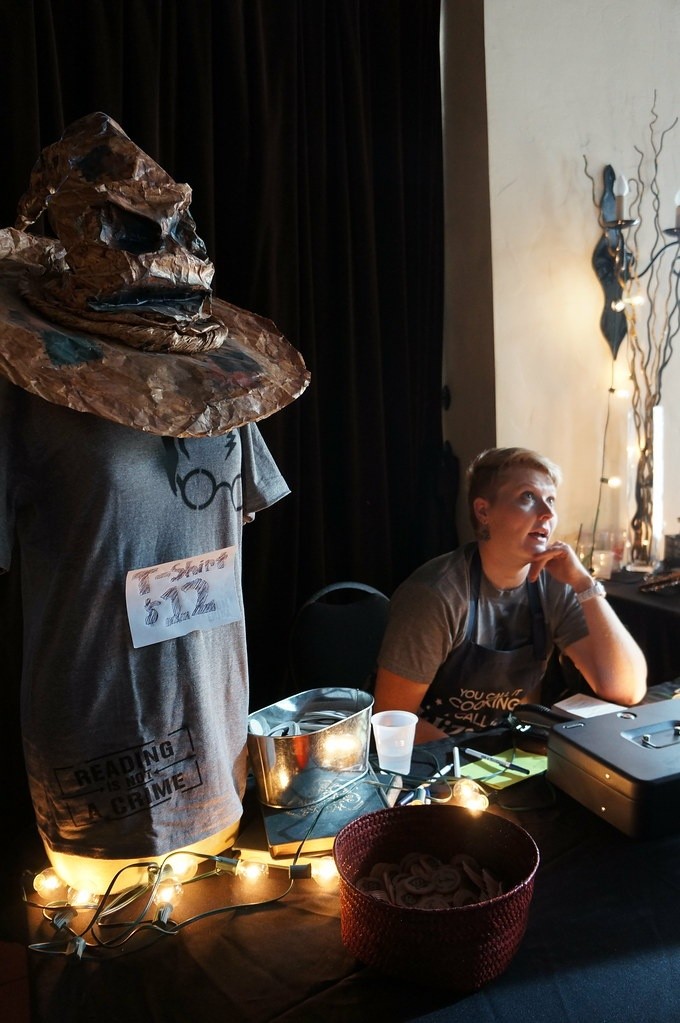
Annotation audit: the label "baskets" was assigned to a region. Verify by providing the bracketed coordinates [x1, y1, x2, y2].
[334, 804, 542, 986]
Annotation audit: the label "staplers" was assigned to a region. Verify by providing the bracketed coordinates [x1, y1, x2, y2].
[513, 702, 583, 755]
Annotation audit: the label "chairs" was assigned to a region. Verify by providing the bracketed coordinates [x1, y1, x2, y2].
[287, 574, 391, 694]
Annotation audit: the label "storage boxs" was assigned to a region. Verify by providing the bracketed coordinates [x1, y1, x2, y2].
[547, 699, 680, 840]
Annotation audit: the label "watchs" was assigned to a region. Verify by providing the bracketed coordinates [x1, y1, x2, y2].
[576, 581, 607, 603]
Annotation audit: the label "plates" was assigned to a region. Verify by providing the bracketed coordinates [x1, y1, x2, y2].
[263, 709, 358, 738]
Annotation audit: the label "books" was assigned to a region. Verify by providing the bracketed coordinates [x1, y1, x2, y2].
[260, 759, 393, 858]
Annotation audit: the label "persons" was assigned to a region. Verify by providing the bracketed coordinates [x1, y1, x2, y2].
[0, 110, 312, 896]
[373, 447, 649, 746]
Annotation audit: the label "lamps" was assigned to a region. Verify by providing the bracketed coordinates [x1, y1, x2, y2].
[589, 162, 680, 359]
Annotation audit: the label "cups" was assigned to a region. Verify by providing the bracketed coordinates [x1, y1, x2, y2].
[370, 710, 418, 776]
[605, 530, 627, 573]
[592, 549, 614, 581]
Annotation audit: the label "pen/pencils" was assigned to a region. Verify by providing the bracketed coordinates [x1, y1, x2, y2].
[458, 747, 530, 775]
[453, 746, 460, 778]
[394, 763, 453, 807]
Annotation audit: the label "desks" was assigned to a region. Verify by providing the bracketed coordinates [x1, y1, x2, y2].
[16, 676, 680, 1023]
[601, 568, 680, 687]
